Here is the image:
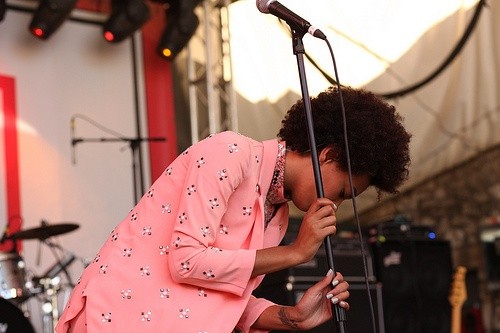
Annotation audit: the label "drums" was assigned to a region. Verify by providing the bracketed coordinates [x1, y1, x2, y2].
[0, 250, 34, 305]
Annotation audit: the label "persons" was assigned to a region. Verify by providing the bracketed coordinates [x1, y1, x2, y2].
[55, 86, 412, 333]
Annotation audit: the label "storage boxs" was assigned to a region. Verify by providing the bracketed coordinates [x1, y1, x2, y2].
[290, 246, 375, 282]
[288, 283, 385, 333]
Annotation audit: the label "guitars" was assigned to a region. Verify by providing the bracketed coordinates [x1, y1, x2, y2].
[448, 266, 467, 333]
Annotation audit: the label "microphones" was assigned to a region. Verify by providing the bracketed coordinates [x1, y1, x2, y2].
[256, 0, 326, 40]
[71, 118, 77, 163]
[0, 224, 11, 243]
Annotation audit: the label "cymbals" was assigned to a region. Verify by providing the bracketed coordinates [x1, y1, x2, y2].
[0, 223, 82, 243]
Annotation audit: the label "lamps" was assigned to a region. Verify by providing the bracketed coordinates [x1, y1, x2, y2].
[157, 0, 207, 61]
[102, 1, 151, 43]
[26, 0, 78, 41]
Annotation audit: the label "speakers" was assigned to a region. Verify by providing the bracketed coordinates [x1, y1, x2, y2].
[372, 239, 456, 333]
